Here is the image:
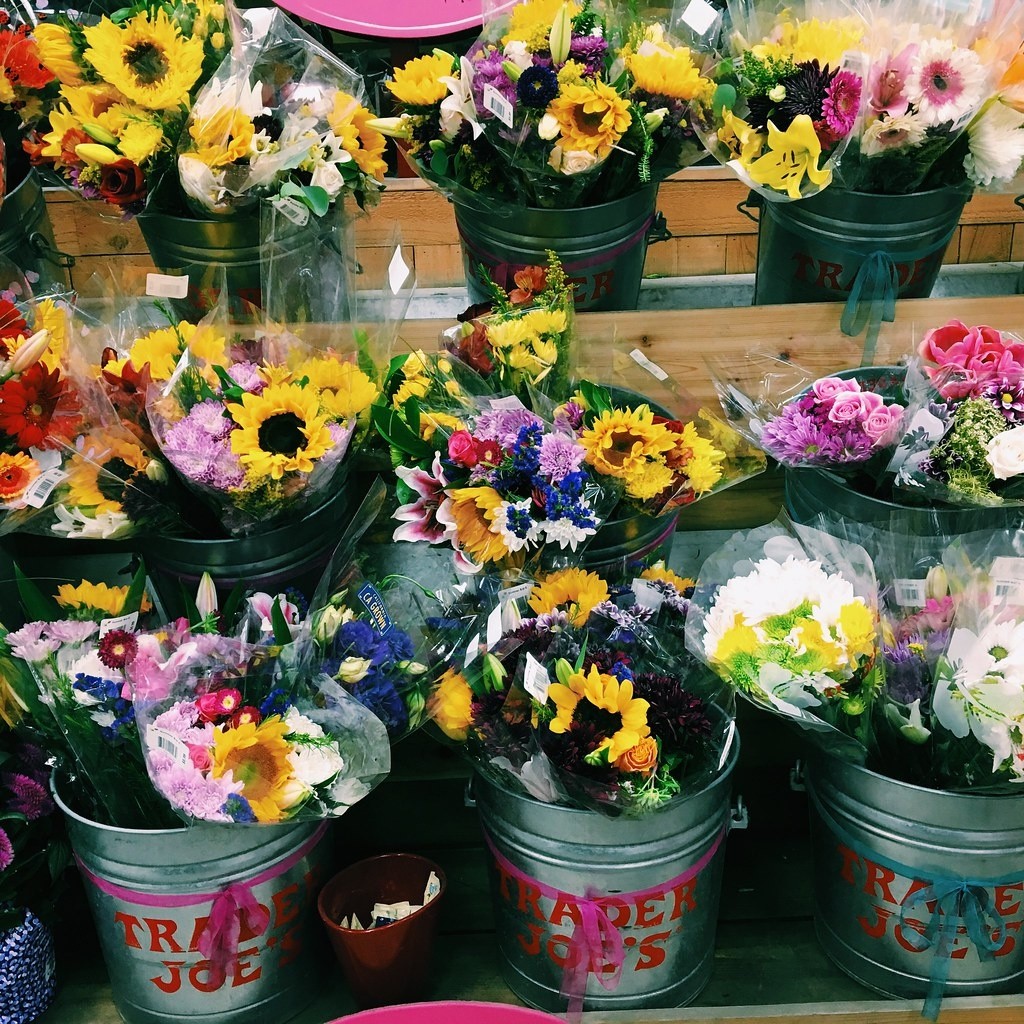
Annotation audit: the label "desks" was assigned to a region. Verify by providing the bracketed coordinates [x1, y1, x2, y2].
[231, 293, 1024, 533]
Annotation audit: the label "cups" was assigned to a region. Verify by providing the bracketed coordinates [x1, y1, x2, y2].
[318, 852, 446, 1009]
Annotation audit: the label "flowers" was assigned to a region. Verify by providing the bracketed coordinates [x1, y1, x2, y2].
[372, 245, 728, 554]
[19, 0, 395, 220]
[415, 555, 703, 813]
[1, 8, 49, 196]
[691, 3, 1023, 209]
[1, 290, 92, 531]
[666, 519, 1024, 788]
[757, 316, 1023, 508]
[4, 567, 411, 827]
[349, 4, 719, 196]
[58, 311, 381, 516]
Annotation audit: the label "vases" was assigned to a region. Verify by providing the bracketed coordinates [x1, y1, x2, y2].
[0, 498, 168, 633]
[126, 459, 353, 621]
[1, 166, 71, 297]
[781, 726, 1024, 998]
[447, 167, 664, 311]
[438, 694, 750, 1005]
[135, 189, 352, 326]
[727, 163, 976, 302]
[43, 745, 331, 1023]
[782, 365, 1023, 579]
[472, 373, 689, 581]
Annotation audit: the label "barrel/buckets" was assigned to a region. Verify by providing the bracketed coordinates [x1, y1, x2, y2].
[736, 181, 976, 304]
[139, 476, 356, 626]
[774, 365, 1023, 610]
[462, 700, 740, 1013]
[469, 381, 679, 588]
[47, 756, 329, 1024]
[443, 180, 673, 312]
[133, 198, 364, 324]
[793, 742, 1024, 1000]
[1, 169, 77, 301]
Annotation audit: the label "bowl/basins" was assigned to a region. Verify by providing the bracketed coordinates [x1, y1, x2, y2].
[323, 1001, 568, 1024]
